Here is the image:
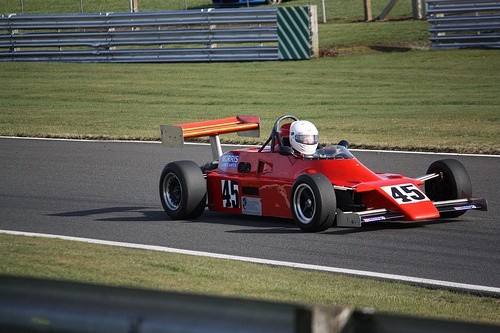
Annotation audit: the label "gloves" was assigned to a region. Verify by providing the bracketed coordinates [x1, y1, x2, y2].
[320, 147, 336, 155]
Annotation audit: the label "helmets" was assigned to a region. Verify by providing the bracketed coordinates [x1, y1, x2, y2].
[289, 121, 319, 155]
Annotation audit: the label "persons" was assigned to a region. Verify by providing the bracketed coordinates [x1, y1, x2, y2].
[289, 120, 323, 155]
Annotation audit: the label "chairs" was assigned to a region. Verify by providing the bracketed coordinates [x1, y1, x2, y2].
[270, 115, 300, 152]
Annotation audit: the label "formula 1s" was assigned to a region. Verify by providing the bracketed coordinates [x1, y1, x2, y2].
[159, 115, 489, 232]
